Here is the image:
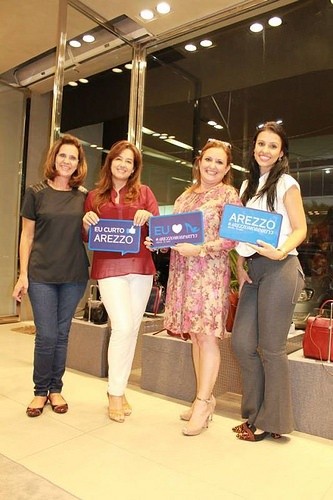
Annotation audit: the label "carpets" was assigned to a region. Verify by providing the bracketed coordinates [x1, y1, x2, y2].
[10, 325, 36, 335]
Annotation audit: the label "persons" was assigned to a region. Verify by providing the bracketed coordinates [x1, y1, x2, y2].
[82, 142, 159, 423]
[144, 138, 242, 435]
[233, 122, 307, 440]
[11, 136, 90, 417]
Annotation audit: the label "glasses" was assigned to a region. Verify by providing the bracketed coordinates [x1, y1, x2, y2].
[207, 138, 231, 149]
[256, 120, 283, 132]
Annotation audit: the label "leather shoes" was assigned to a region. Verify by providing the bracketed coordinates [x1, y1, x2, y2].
[26, 396, 50, 417]
[48, 393, 68, 413]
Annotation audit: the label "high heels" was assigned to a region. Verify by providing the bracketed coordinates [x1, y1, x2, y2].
[122, 394, 132, 416]
[236, 424, 281, 441]
[232, 419, 254, 433]
[183, 398, 213, 436]
[180, 392, 216, 421]
[107, 391, 125, 422]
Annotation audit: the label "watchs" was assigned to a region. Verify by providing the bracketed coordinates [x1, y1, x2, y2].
[278, 248, 288, 261]
[199, 248, 206, 257]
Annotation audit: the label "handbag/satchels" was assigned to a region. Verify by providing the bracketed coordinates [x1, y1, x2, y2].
[225, 289, 240, 332]
[303, 300, 333, 361]
[145, 273, 166, 314]
[154, 328, 190, 340]
[83, 285, 108, 323]
